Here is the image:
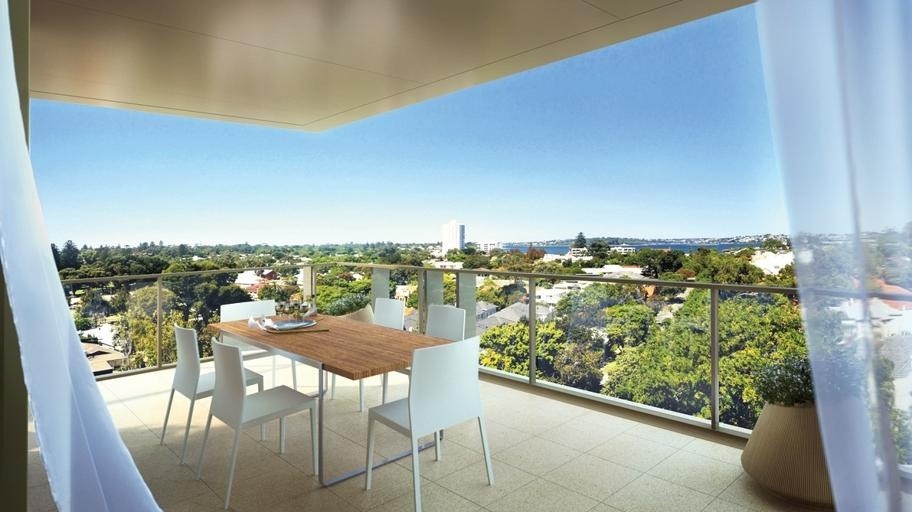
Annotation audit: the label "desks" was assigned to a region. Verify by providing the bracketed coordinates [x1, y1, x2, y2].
[207, 312, 455, 486]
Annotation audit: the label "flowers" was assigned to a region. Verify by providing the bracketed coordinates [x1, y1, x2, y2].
[749, 351, 821, 407]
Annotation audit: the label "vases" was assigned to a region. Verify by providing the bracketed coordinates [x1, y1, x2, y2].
[737, 402, 839, 507]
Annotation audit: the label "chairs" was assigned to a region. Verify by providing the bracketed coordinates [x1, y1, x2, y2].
[366, 335, 496, 512]
[161, 320, 264, 467]
[219, 299, 296, 392]
[382, 303, 466, 448]
[331, 298, 406, 414]
[195, 337, 318, 510]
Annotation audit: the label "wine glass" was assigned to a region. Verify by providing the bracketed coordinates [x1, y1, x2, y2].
[274, 300, 311, 323]
